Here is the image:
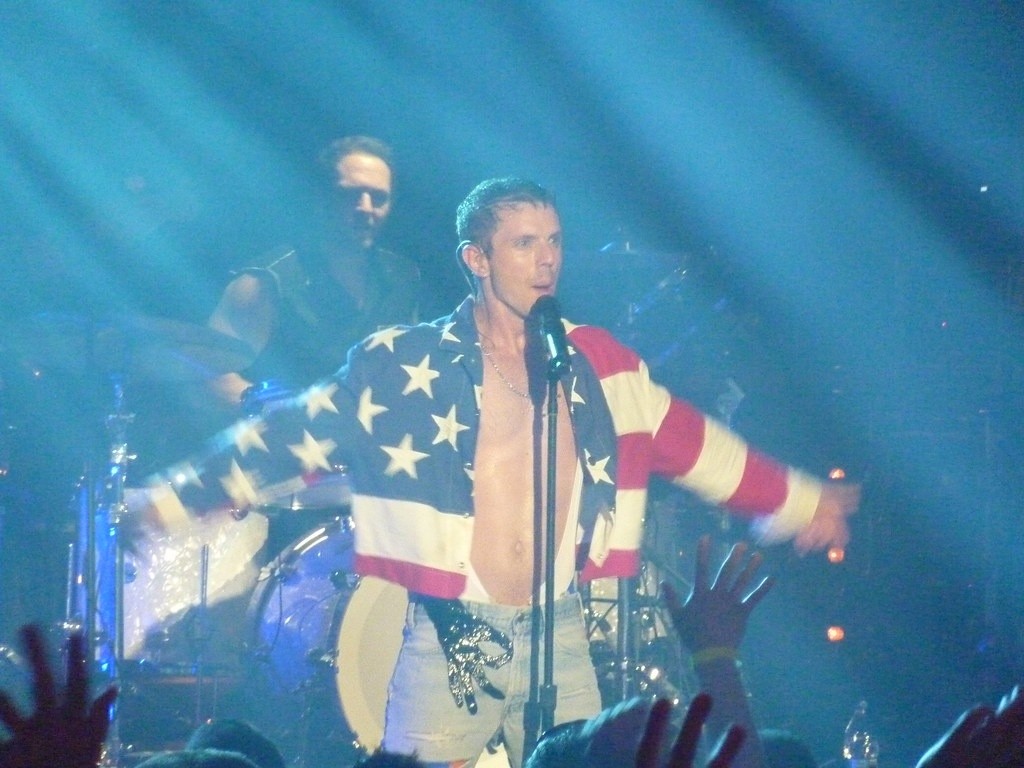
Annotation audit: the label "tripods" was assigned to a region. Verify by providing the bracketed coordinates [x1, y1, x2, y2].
[592, 581, 694, 717]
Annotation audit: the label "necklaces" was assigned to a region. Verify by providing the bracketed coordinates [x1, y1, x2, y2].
[481, 342, 530, 398]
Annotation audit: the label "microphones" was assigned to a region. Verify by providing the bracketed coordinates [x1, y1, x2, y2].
[534, 293, 570, 375]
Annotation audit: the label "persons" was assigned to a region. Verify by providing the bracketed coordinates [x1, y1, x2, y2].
[0, 535, 1024, 768]
[204, 135, 452, 423]
[116, 176, 859, 768]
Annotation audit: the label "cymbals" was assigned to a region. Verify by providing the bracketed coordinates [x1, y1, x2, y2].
[3, 311, 257, 382]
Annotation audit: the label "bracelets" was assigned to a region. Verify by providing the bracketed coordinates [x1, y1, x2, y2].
[690, 645, 739, 669]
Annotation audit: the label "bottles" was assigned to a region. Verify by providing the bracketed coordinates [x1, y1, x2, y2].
[843, 698, 881, 768]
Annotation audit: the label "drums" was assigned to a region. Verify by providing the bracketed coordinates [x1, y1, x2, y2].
[242, 513, 410, 768]
[73, 486, 283, 686]
[239, 373, 354, 510]
